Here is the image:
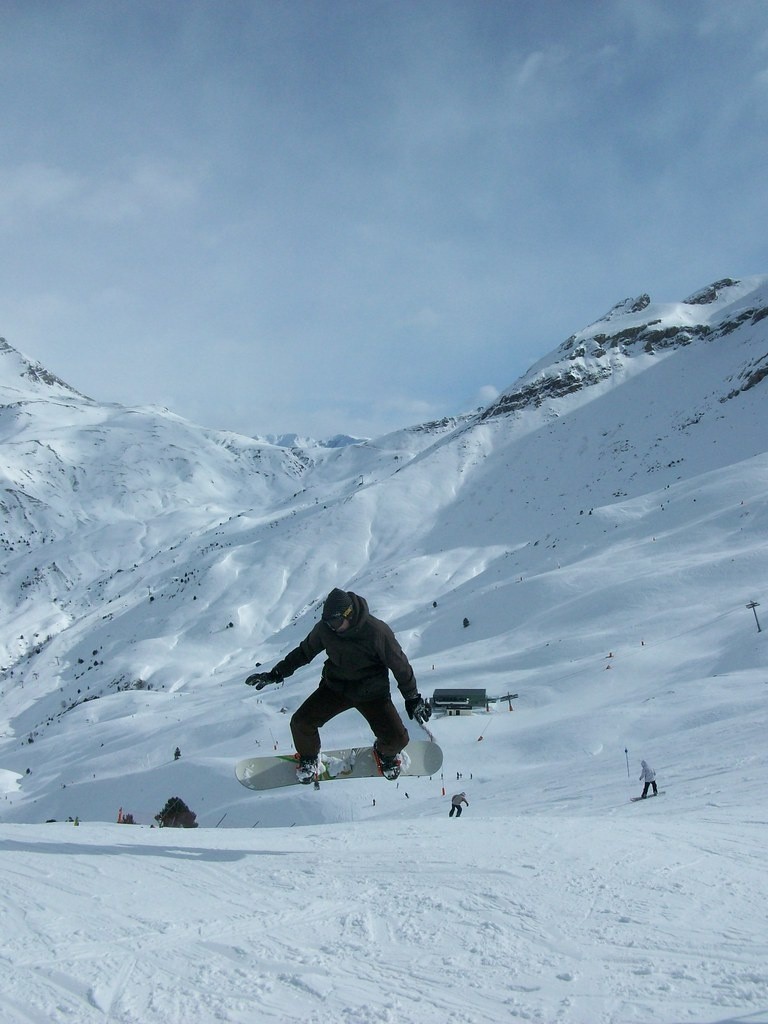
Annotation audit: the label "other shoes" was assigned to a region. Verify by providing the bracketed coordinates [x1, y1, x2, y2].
[295, 758, 319, 784]
[372, 741, 402, 780]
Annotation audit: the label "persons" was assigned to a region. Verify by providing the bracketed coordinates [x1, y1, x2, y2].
[639, 760, 658, 799]
[405, 792, 409, 798]
[449, 792, 469, 817]
[245, 587, 432, 785]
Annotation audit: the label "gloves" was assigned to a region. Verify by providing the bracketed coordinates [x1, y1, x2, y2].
[402, 688, 432, 725]
[245, 668, 285, 692]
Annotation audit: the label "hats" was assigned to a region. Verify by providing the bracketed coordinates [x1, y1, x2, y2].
[321, 588, 353, 620]
[461, 791, 467, 797]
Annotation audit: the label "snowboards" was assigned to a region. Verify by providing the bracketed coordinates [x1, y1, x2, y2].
[233, 740, 443, 790]
[630, 791, 666, 802]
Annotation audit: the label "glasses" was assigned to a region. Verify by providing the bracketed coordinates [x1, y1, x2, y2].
[321, 614, 345, 629]
